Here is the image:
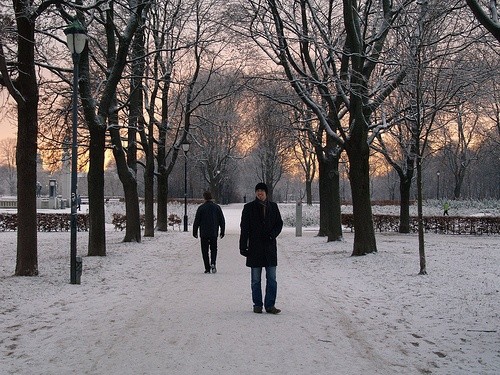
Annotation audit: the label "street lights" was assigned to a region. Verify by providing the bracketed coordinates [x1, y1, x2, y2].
[180, 140, 192, 235]
[63, 14, 89, 285]
[436, 172, 440, 200]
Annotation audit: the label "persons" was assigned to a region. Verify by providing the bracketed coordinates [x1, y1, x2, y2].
[239, 182, 283, 314]
[192, 190, 225, 274]
[77, 195, 81, 211]
[36, 181, 42, 198]
[443, 201, 449, 216]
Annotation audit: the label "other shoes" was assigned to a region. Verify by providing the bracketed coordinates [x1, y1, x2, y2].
[205, 269, 210, 273]
[254, 307, 262, 313]
[267, 307, 281, 314]
[211, 264, 216, 273]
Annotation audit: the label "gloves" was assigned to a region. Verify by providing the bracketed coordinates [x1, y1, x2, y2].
[193, 234, 198, 238]
[240, 249, 248, 257]
[220, 233, 224, 239]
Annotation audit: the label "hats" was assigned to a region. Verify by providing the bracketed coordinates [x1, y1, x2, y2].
[255, 182, 268, 195]
